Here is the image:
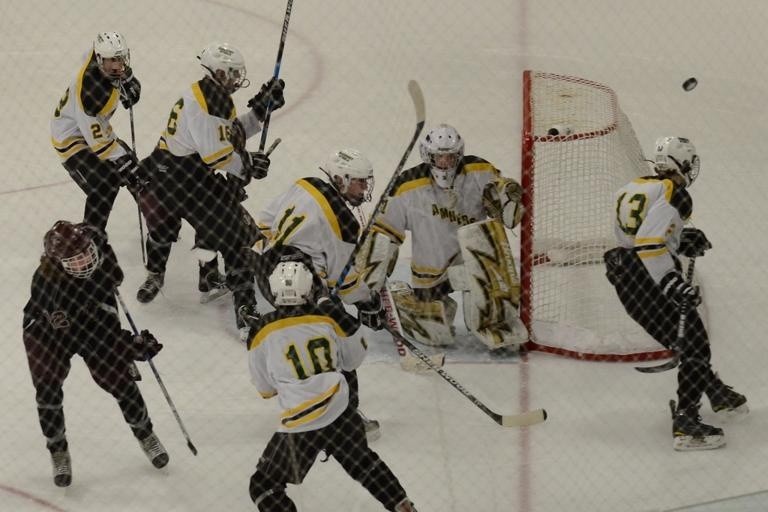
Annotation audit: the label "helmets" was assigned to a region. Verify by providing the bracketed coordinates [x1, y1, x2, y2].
[654, 137, 699, 187]
[201, 43, 245, 94]
[95, 31, 129, 77]
[269, 261, 313, 306]
[44, 221, 99, 279]
[331, 149, 374, 206]
[420, 125, 464, 189]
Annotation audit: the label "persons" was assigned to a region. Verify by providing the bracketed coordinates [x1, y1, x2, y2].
[603, 134, 747, 453]
[20, 220, 171, 488]
[245, 259, 417, 511]
[128, 118, 253, 303]
[136, 44, 287, 333]
[240, 149, 387, 343]
[359, 123, 524, 369]
[50, 29, 181, 248]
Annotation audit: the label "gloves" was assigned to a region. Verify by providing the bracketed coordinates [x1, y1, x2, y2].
[679, 228, 711, 257]
[247, 78, 285, 122]
[120, 68, 140, 109]
[110, 155, 150, 194]
[353, 293, 386, 331]
[240, 151, 270, 179]
[129, 329, 161, 361]
[660, 272, 701, 310]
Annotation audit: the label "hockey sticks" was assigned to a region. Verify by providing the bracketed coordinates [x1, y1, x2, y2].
[381, 320, 547, 427]
[634, 257, 694, 373]
[113, 285, 198, 455]
[330, 80, 425, 296]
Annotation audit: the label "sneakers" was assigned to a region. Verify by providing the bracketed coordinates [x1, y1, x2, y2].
[706, 378, 746, 412]
[52, 451, 70, 487]
[139, 432, 169, 468]
[137, 273, 163, 302]
[673, 414, 723, 437]
[199, 265, 264, 329]
